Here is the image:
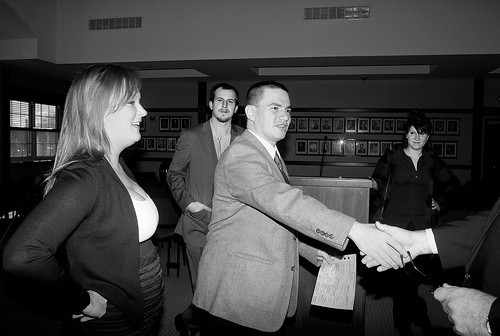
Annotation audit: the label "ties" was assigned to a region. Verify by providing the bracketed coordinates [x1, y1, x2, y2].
[274, 151, 292, 186]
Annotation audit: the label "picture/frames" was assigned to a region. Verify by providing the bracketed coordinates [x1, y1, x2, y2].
[287, 115, 462, 137]
[294, 138, 458, 159]
[159, 115, 192, 133]
[124, 136, 180, 153]
[139, 117, 146, 132]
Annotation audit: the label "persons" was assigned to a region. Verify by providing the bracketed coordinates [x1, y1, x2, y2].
[190, 80, 412, 336]
[165, 83, 244, 336]
[367, 110, 463, 336]
[359, 189, 500, 336]
[121, 115, 465, 157]
[2, 63, 168, 336]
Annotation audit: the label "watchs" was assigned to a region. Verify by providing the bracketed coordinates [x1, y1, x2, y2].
[485, 317, 493, 335]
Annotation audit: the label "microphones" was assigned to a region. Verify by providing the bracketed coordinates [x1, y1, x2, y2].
[320, 136, 327, 176]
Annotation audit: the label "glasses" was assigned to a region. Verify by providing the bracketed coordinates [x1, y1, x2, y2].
[400, 251, 442, 295]
